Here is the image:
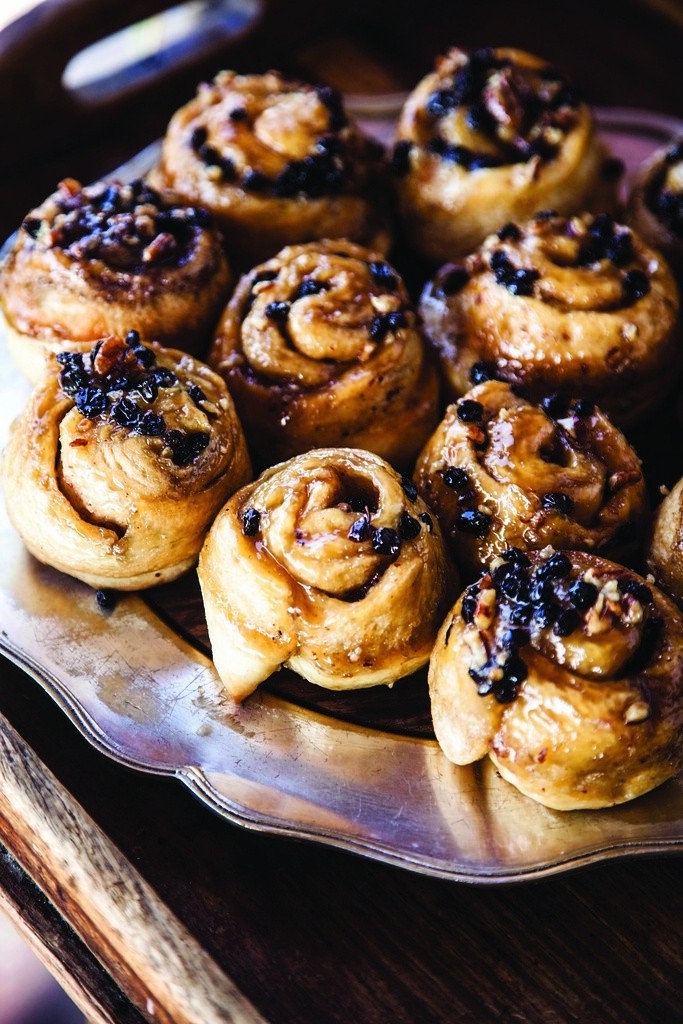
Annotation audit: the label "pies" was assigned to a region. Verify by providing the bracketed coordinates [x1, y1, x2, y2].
[2, 39, 683, 810]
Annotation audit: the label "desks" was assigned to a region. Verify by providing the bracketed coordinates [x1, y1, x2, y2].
[0, 0, 682, 1024]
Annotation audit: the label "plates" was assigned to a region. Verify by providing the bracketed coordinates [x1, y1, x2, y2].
[0, 97, 683, 885]
